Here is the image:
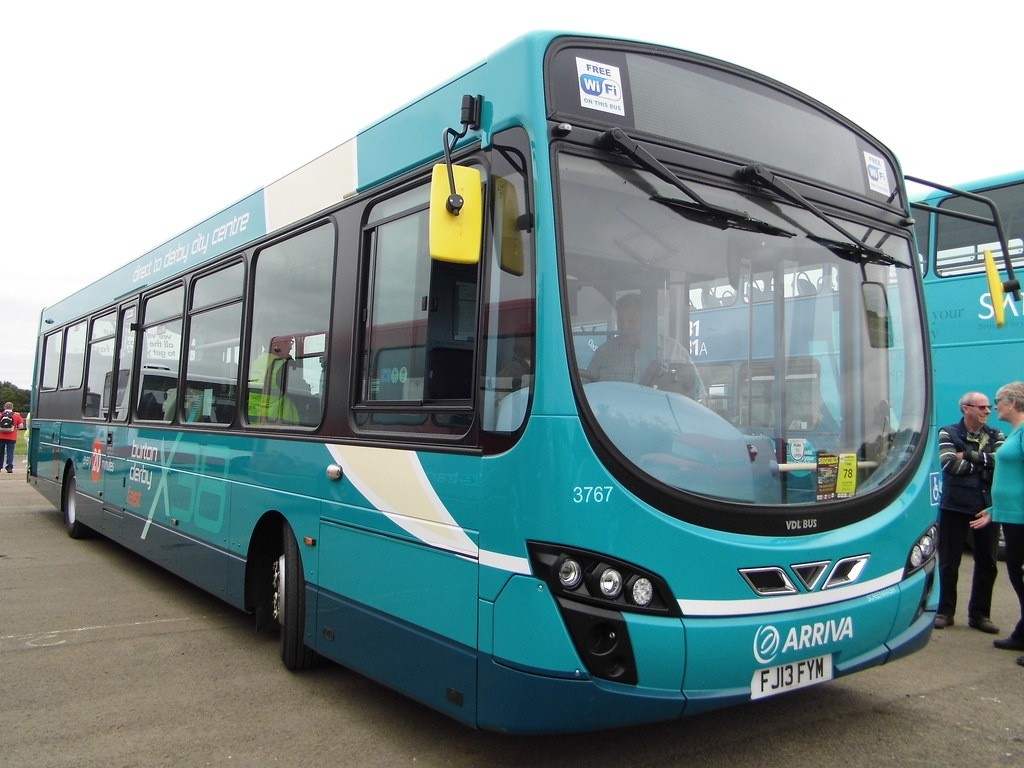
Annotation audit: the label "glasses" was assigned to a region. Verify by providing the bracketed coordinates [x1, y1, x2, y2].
[277, 340, 294, 345]
[964, 404, 992, 411]
[994, 399, 1003, 405]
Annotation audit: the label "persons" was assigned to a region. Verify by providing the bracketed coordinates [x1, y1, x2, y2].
[138, 379, 202, 422]
[23, 411, 30, 468]
[247, 321, 313, 427]
[0, 402, 24, 474]
[968, 381, 1024, 667]
[933, 390, 1007, 634]
[811, 400, 843, 451]
[493, 323, 532, 406]
[582, 293, 708, 408]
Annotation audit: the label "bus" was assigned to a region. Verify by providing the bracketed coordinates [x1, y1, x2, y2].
[258, 297, 534, 457]
[98, 362, 316, 430]
[21, 25, 1023, 737]
[572, 168, 1023, 559]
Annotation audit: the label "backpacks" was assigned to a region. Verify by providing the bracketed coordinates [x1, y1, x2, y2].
[0, 412, 17, 432]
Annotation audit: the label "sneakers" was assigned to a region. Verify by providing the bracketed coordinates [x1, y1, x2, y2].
[969, 617, 1000, 634]
[933, 614, 954, 629]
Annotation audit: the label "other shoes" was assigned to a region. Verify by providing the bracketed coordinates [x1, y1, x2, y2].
[993, 637, 1024, 651]
[7, 468, 12, 473]
[1017, 656, 1024, 666]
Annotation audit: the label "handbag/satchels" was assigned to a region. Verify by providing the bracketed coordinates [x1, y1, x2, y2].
[649, 364, 695, 401]
